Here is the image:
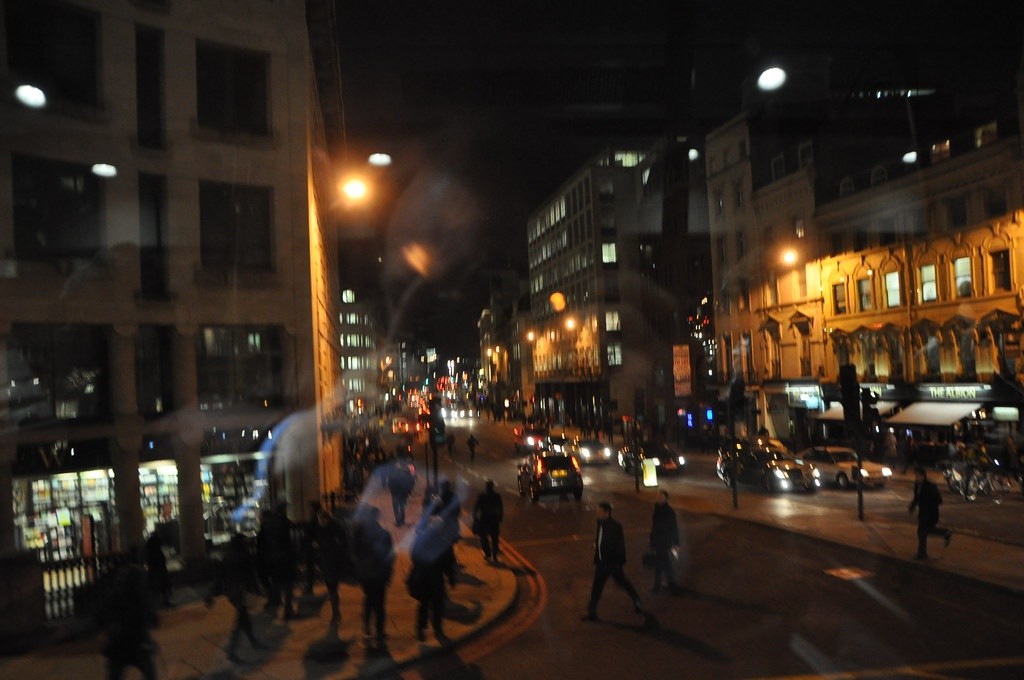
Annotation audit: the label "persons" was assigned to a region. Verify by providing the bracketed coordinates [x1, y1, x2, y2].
[870, 424, 1024, 493]
[202, 501, 348, 665]
[97, 580, 155, 680]
[910, 467, 952, 561]
[467, 434, 479, 460]
[487, 401, 526, 425]
[447, 432, 457, 458]
[582, 502, 643, 619]
[578, 413, 614, 443]
[408, 479, 461, 641]
[350, 508, 393, 641]
[523, 409, 555, 433]
[390, 463, 410, 526]
[473, 479, 503, 563]
[646, 490, 680, 595]
[352, 446, 411, 487]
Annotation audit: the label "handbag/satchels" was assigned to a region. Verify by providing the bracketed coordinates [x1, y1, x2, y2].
[471, 518, 481, 533]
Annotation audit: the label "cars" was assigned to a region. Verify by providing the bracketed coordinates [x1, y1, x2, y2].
[575, 439, 611, 465]
[797, 446, 892, 489]
[514, 427, 549, 446]
[618, 441, 686, 475]
[717, 437, 820, 494]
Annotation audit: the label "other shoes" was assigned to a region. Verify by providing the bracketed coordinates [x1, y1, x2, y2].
[944, 529, 952, 547]
[634, 598, 643, 613]
[914, 553, 928, 561]
[435, 631, 449, 642]
[582, 612, 598, 620]
[417, 627, 424, 642]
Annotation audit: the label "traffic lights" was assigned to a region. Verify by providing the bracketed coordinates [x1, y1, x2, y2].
[434, 420, 445, 446]
[839, 364, 859, 401]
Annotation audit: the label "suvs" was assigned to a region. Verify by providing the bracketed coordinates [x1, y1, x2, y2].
[516, 450, 583, 502]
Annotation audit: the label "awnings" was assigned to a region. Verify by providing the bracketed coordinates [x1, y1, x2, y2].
[816, 401, 898, 421]
[882, 401, 982, 426]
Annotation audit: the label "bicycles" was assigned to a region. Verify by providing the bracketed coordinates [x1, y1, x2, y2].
[946, 462, 1011, 504]
